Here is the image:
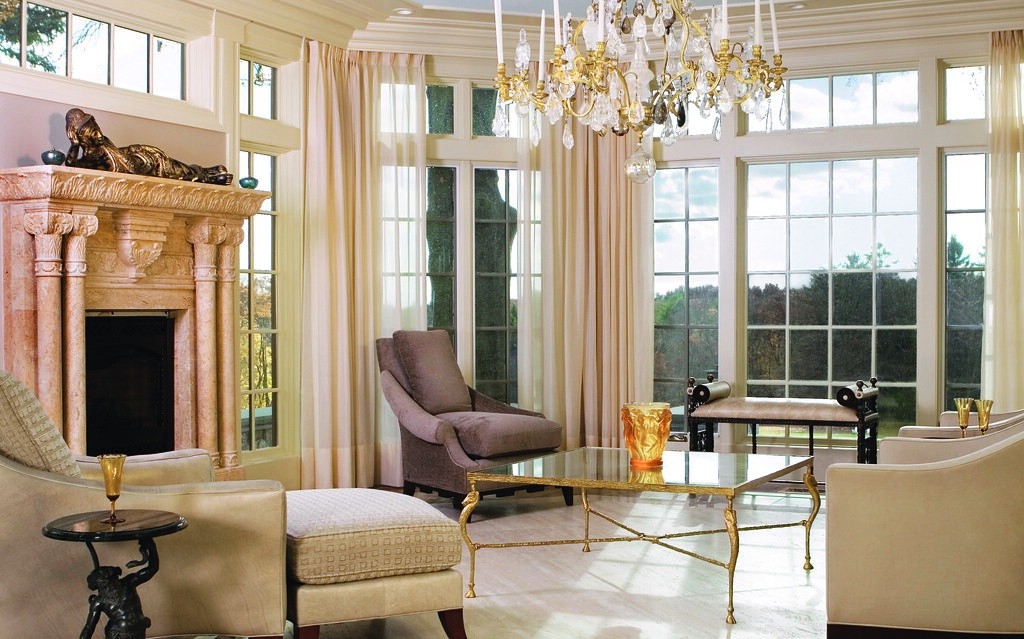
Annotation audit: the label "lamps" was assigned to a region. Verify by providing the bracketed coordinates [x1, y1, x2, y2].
[493, 0, 788, 184]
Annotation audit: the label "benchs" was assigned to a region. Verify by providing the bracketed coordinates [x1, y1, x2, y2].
[689, 375, 878, 485]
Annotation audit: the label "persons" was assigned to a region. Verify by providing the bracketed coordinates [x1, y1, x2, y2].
[65, 108, 233, 185]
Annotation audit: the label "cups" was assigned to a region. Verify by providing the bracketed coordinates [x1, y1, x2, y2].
[622, 400, 673, 468]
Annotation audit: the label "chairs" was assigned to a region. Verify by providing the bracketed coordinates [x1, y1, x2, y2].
[376, 330, 574, 523]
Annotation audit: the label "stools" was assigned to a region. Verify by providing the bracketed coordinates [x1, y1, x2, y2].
[286, 488, 467, 639]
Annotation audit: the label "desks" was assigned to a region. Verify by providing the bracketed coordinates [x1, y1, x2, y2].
[42, 509, 188, 639]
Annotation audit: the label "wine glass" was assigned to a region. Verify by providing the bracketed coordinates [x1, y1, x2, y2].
[953, 398, 974, 438]
[97, 454, 127, 523]
[975, 400, 994, 435]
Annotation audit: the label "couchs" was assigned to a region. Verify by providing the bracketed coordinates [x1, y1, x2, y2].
[0, 373, 287, 639]
[827, 409, 1024, 639]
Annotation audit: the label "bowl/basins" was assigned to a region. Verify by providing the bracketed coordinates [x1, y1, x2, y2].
[41, 148, 65, 164]
[240, 176, 258, 189]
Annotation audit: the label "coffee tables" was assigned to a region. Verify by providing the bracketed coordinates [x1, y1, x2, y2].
[460, 447, 821, 623]
